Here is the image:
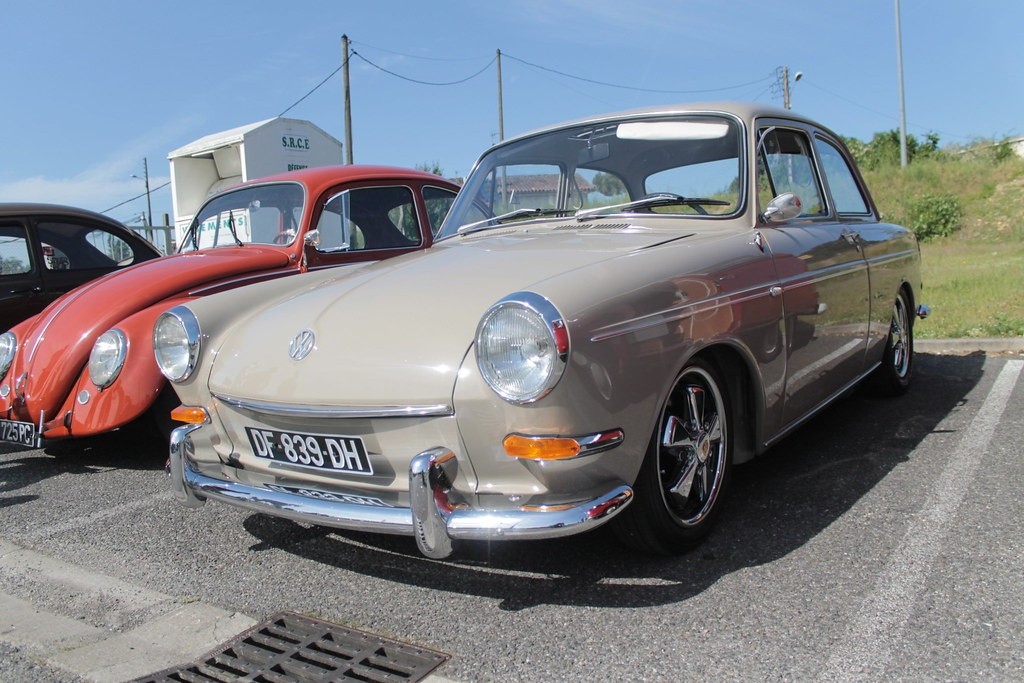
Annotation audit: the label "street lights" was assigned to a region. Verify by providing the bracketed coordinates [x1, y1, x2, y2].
[781, 65, 803, 111]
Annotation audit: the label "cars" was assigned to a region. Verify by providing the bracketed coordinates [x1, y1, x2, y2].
[0, 201, 166, 332]
[0, 163, 501, 443]
[148, 102, 931, 560]
